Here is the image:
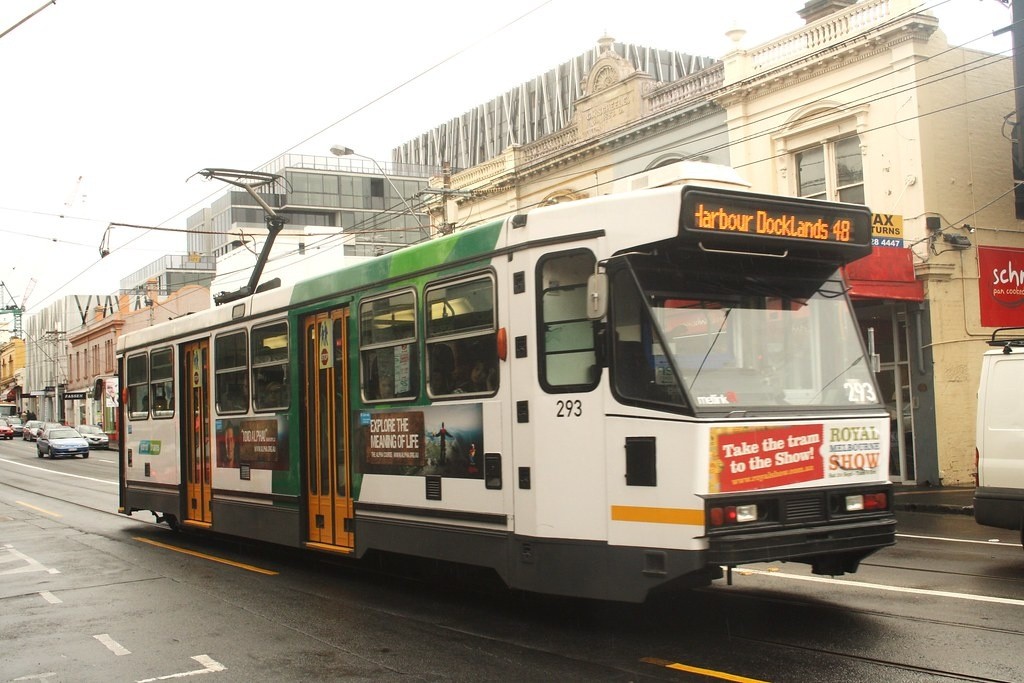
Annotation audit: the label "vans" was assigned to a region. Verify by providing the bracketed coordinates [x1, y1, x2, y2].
[972, 328, 1024, 555]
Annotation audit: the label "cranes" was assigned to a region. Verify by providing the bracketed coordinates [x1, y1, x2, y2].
[0, 278, 38, 341]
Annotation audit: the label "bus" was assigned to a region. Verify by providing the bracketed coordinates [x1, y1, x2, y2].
[115, 166, 900, 635]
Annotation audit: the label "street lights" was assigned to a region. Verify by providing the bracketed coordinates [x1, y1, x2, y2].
[331, 143, 434, 240]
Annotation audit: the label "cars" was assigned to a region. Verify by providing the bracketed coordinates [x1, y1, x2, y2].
[0, 419, 14, 441]
[75, 425, 110, 450]
[22, 420, 45, 442]
[36, 429, 90, 460]
[6, 418, 23, 437]
[37, 422, 63, 437]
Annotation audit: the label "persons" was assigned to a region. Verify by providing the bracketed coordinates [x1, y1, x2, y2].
[433, 423, 453, 463]
[451, 361, 497, 394]
[17, 410, 36, 422]
[468, 443, 476, 463]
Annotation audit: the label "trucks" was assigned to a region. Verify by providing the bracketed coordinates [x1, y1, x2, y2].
[0, 404, 20, 420]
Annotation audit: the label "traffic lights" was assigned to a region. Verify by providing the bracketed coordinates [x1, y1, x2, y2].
[94, 379, 103, 400]
